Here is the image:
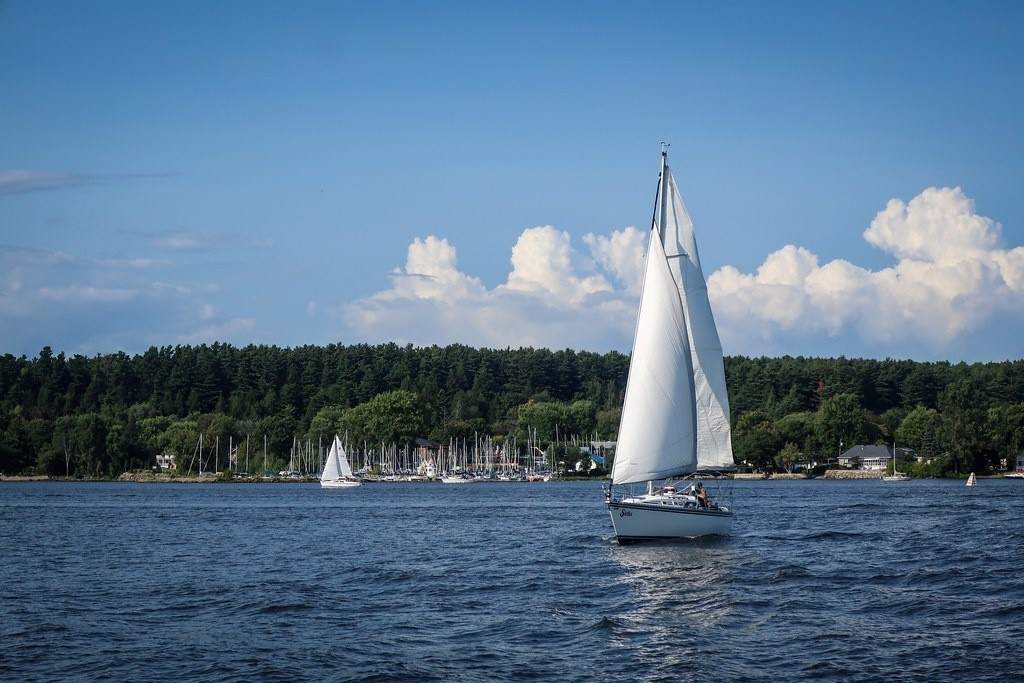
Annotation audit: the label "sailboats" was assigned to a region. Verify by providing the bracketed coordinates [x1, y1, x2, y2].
[317, 434, 362, 487]
[881, 441, 911, 482]
[964, 471, 978, 487]
[186, 424, 609, 483]
[601, 138, 739, 545]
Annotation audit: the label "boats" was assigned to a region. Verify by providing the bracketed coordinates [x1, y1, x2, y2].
[1003, 473, 1024, 479]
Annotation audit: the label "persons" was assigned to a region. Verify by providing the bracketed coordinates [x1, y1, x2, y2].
[698, 488, 712, 509]
[697, 482, 707, 499]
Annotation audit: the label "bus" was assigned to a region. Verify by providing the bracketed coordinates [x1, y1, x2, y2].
[150, 455, 178, 471]
